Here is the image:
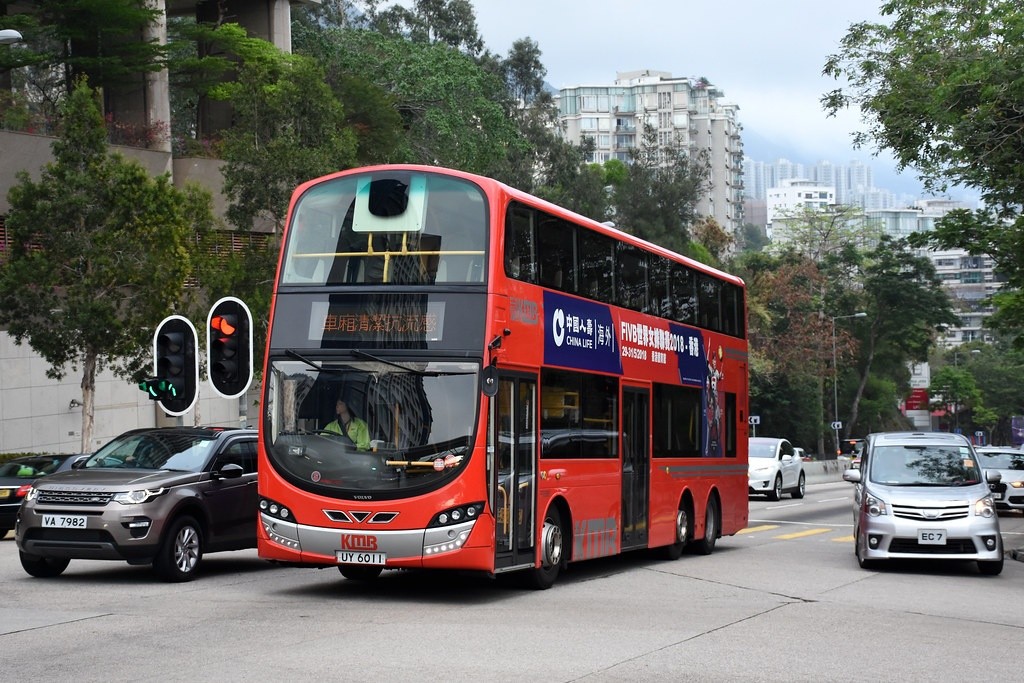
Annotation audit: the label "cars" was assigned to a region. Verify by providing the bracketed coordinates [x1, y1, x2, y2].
[0, 453, 126, 538]
[840, 432, 1005, 576]
[973, 443, 1024, 513]
[747, 437, 813, 500]
[16, 426, 258, 585]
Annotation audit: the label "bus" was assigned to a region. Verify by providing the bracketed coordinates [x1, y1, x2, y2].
[257, 163, 750, 590]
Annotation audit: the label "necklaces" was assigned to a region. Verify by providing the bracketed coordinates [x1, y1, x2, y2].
[344, 419, 351, 427]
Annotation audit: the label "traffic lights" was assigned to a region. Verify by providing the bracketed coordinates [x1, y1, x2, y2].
[206, 297, 253, 399]
[140, 314, 200, 415]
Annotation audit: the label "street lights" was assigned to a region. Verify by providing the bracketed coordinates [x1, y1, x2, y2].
[832, 313, 868, 458]
[954, 350, 981, 432]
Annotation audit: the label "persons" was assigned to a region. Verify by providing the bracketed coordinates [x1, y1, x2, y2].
[318, 393, 372, 452]
